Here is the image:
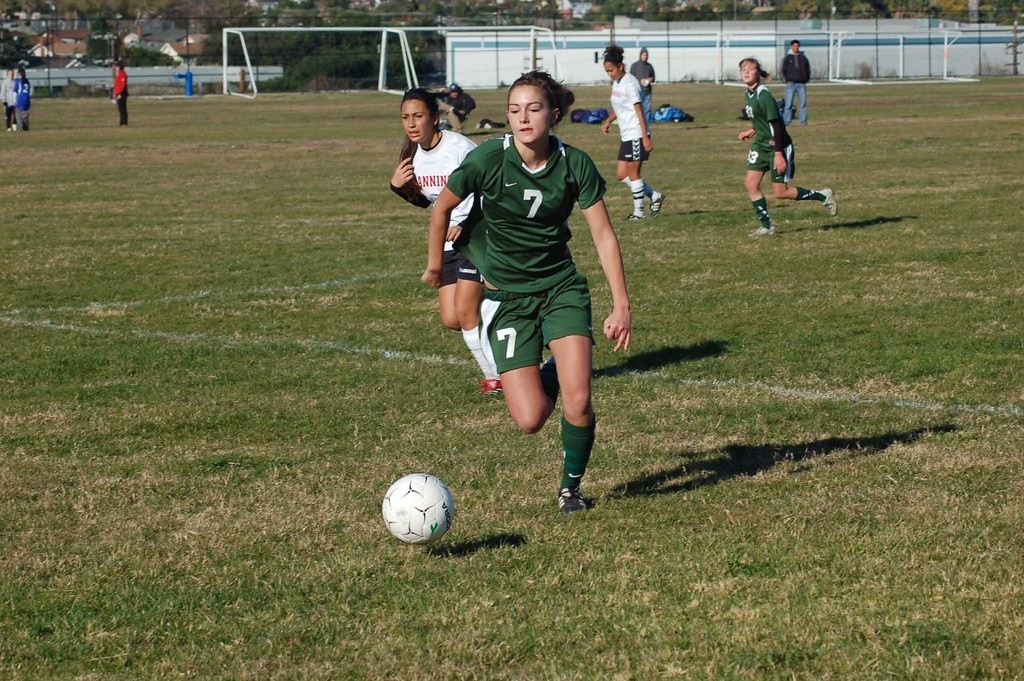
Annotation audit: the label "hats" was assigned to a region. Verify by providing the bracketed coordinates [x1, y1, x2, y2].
[449, 84, 458, 92]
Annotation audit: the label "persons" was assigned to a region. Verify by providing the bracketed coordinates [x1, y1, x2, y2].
[439, 83, 476, 133]
[602, 46, 666, 221]
[630, 47, 655, 124]
[390, 89, 503, 395]
[0, 68, 30, 132]
[780, 40, 810, 125]
[113, 63, 128, 127]
[739, 58, 838, 237]
[421, 72, 631, 513]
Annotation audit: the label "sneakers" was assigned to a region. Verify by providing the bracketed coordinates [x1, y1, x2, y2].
[816, 187, 838, 216]
[557, 485, 586, 514]
[621, 214, 647, 220]
[479, 378, 502, 397]
[748, 224, 775, 238]
[649, 190, 665, 219]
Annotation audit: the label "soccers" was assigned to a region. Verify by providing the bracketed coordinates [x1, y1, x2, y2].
[381, 472, 457, 546]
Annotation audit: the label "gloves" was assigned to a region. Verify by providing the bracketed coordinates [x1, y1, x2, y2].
[452, 108, 459, 113]
[3, 102, 8, 106]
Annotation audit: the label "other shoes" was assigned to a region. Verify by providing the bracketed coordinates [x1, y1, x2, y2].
[6, 128, 11, 132]
[13, 124, 18, 131]
